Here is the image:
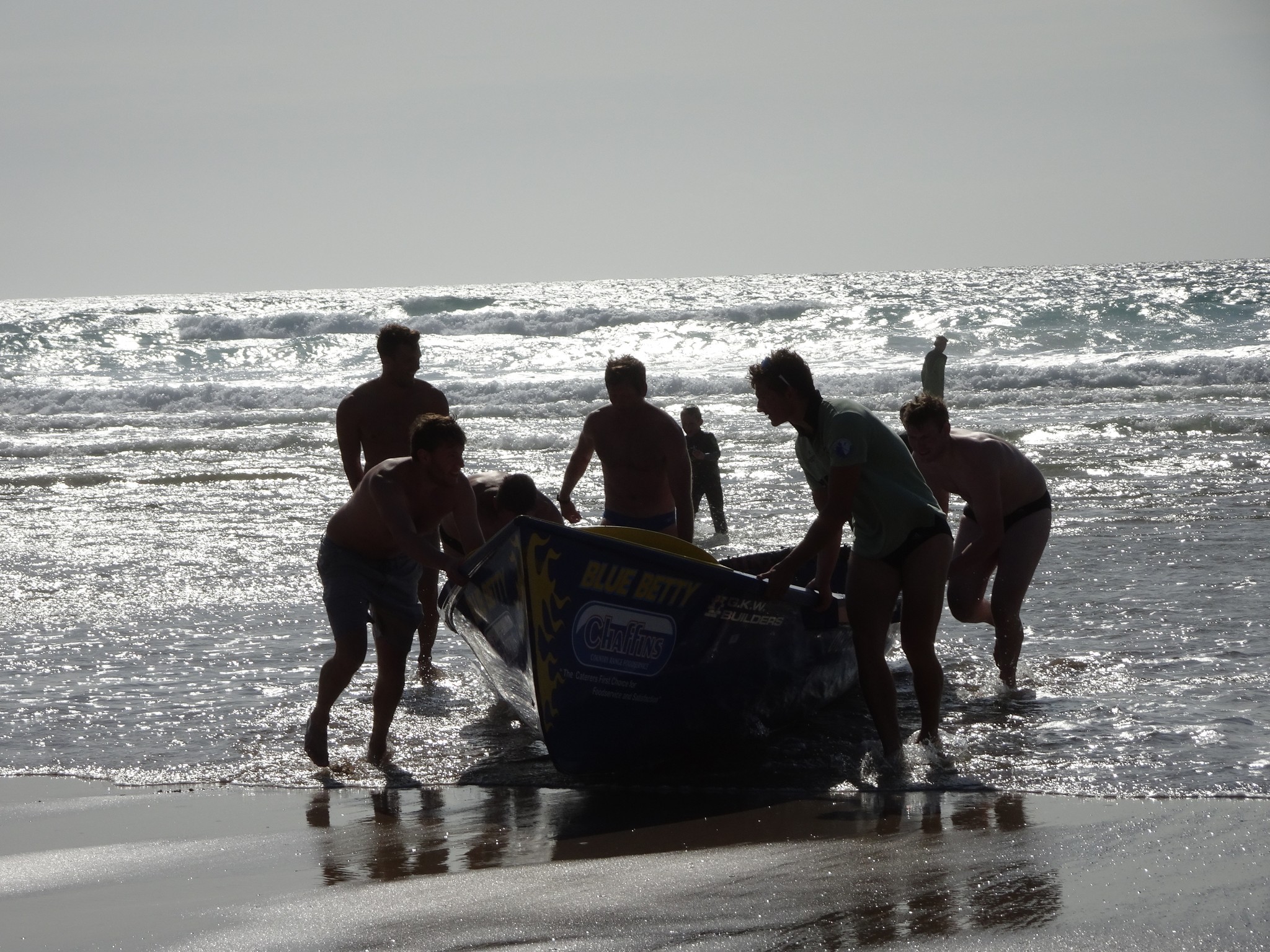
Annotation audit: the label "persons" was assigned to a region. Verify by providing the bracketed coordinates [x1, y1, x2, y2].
[746, 348, 955, 759]
[557, 354, 694, 543]
[920, 335, 948, 400]
[336, 324, 449, 671]
[899, 394, 1053, 685]
[438, 472, 565, 558]
[304, 411, 488, 768]
[680, 404, 728, 535]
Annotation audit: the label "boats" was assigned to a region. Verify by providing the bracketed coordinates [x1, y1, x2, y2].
[434, 512, 911, 795]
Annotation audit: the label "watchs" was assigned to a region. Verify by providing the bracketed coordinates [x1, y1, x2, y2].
[557, 494, 570, 504]
